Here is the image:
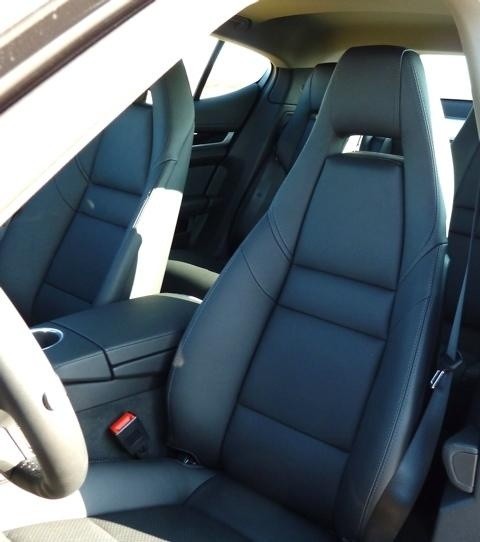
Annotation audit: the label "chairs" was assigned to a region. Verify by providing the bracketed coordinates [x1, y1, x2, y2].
[4, 44, 479, 542]
